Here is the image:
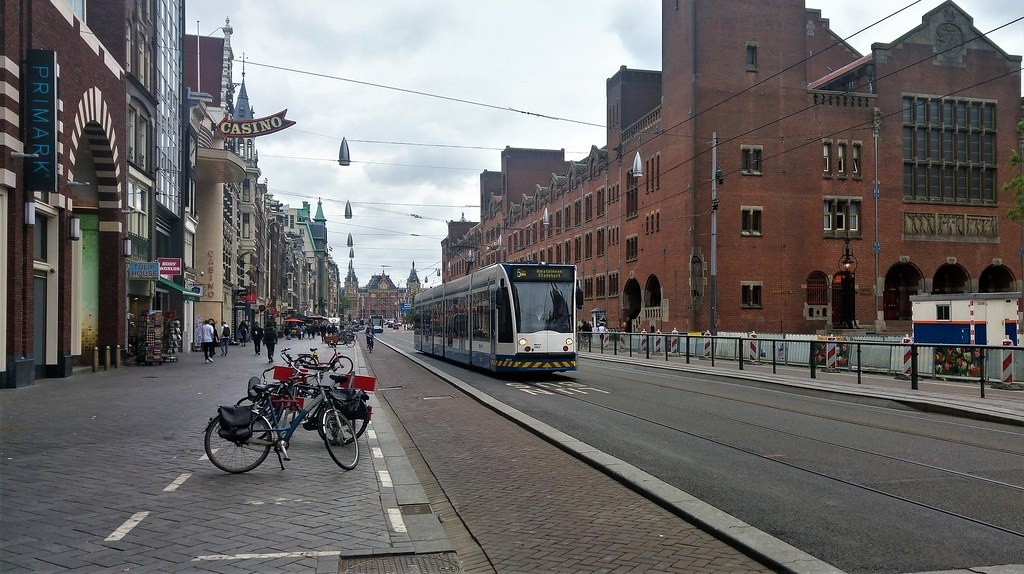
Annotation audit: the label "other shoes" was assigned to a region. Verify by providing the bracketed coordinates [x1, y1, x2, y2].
[221, 352, 226, 356]
[270, 356, 273, 362]
[208, 357, 213, 362]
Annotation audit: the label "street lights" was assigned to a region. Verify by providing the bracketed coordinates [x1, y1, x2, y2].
[837, 229, 860, 329]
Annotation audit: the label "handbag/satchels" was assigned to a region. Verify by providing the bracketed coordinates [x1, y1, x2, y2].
[223, 326, 230, 337]
[218, 405, 253, 441]
[331, 389, 368, 420]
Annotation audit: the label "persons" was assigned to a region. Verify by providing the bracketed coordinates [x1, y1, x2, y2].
[579, 319, 606, 352]
[533, 305, 548, 329]
[202, 319, 345, 363]
[365, 323, 371, 351]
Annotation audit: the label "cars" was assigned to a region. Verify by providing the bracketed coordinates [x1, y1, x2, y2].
[385, 319, 401, 330]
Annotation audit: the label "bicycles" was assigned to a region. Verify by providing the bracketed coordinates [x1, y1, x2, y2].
[201, 347, 372, 474]
[324, 322, 359, 349]
[293, 343, 354, 375]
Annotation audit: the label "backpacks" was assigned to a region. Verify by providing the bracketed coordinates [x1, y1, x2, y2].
[265, 329, 274, 341]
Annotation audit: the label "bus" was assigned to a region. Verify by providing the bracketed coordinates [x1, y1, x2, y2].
[369, 315, 383, 333]
[411, 245, 585, 378]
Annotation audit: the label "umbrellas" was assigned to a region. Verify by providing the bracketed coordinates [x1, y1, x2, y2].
[285, 317, 305, 326]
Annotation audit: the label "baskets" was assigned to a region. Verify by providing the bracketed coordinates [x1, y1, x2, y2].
[247, 376, 261, 401]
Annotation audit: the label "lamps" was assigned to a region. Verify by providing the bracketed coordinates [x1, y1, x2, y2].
[448, 262, 451, 271]
[498, 235, 502, 248]
[339, 137, 350, 165]
[437, 269, 440, 276]
[350, 248, 354, 258]
[468, 249, 472, 260]
[543, 207, 550, 224]
[122, 235, 132, 257]
[347, 233, 353, 247]
[633, 150, 643, 177]
[26, 199, 35, 226]
[345, 200, 352, 219]
[68, 216, 80, 241]
[349, 260, 353, 284]
